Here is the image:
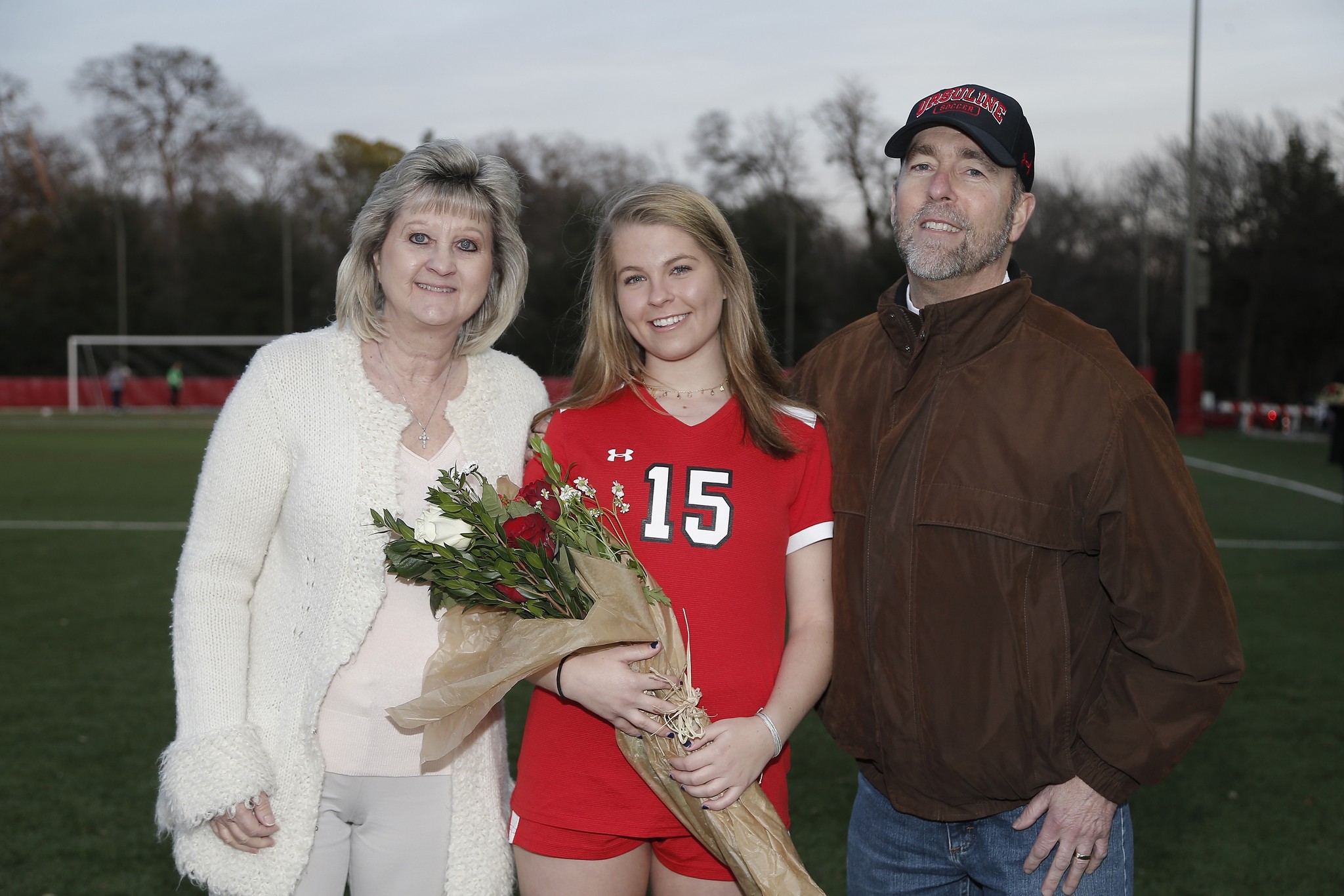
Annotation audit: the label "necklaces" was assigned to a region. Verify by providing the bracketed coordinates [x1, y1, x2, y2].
[641, 369, 729, 400]
[377, 341, 453, 449]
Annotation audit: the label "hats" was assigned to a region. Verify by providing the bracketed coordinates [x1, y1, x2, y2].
[884, 83, 1035, 194]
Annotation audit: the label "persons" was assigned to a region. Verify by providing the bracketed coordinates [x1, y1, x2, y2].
[105, 358, 131, 408]
[156, 139, 551, 896]
[785, 84, 1244, 896]
[166, 360, 184, 408]
[492, 180, 833, 896]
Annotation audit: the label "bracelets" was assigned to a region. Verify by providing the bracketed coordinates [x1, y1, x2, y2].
[754, 707, 782, 784]
[556, 655, 572, 699]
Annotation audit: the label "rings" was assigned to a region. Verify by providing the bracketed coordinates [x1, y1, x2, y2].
[1073, 851, 1091, 860]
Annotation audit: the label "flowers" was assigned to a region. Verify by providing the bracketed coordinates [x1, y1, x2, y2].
[363, 431, 832, 896]
